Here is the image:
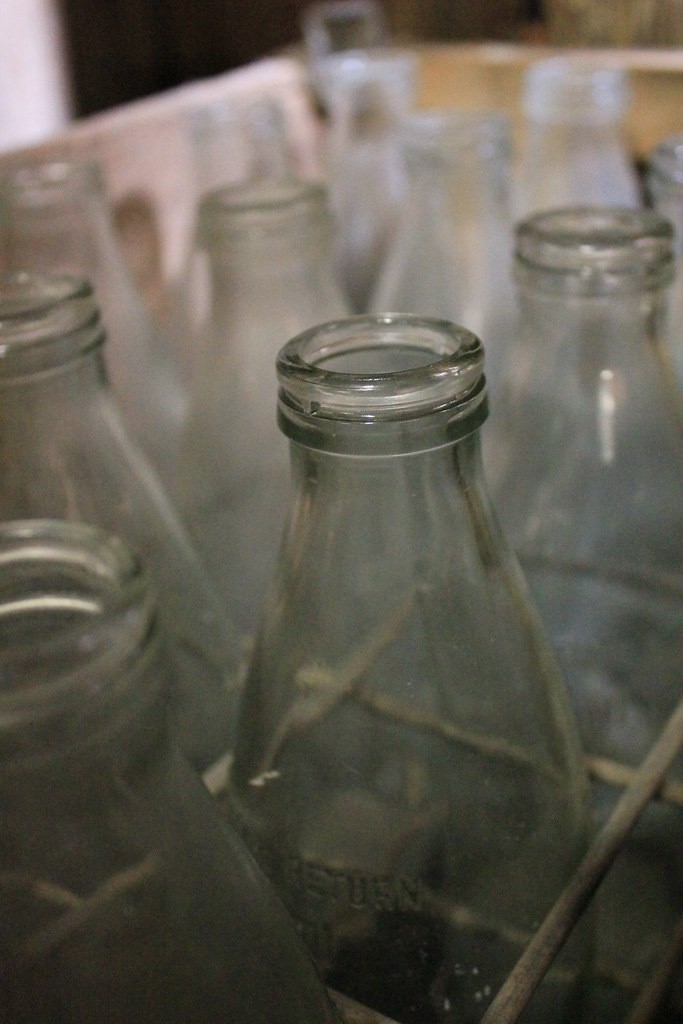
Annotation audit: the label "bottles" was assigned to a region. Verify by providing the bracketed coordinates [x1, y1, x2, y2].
[0, 4, 683, 1024]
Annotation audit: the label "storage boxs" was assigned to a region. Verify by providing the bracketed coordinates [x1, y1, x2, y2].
[3, 46, 682, 1023]
